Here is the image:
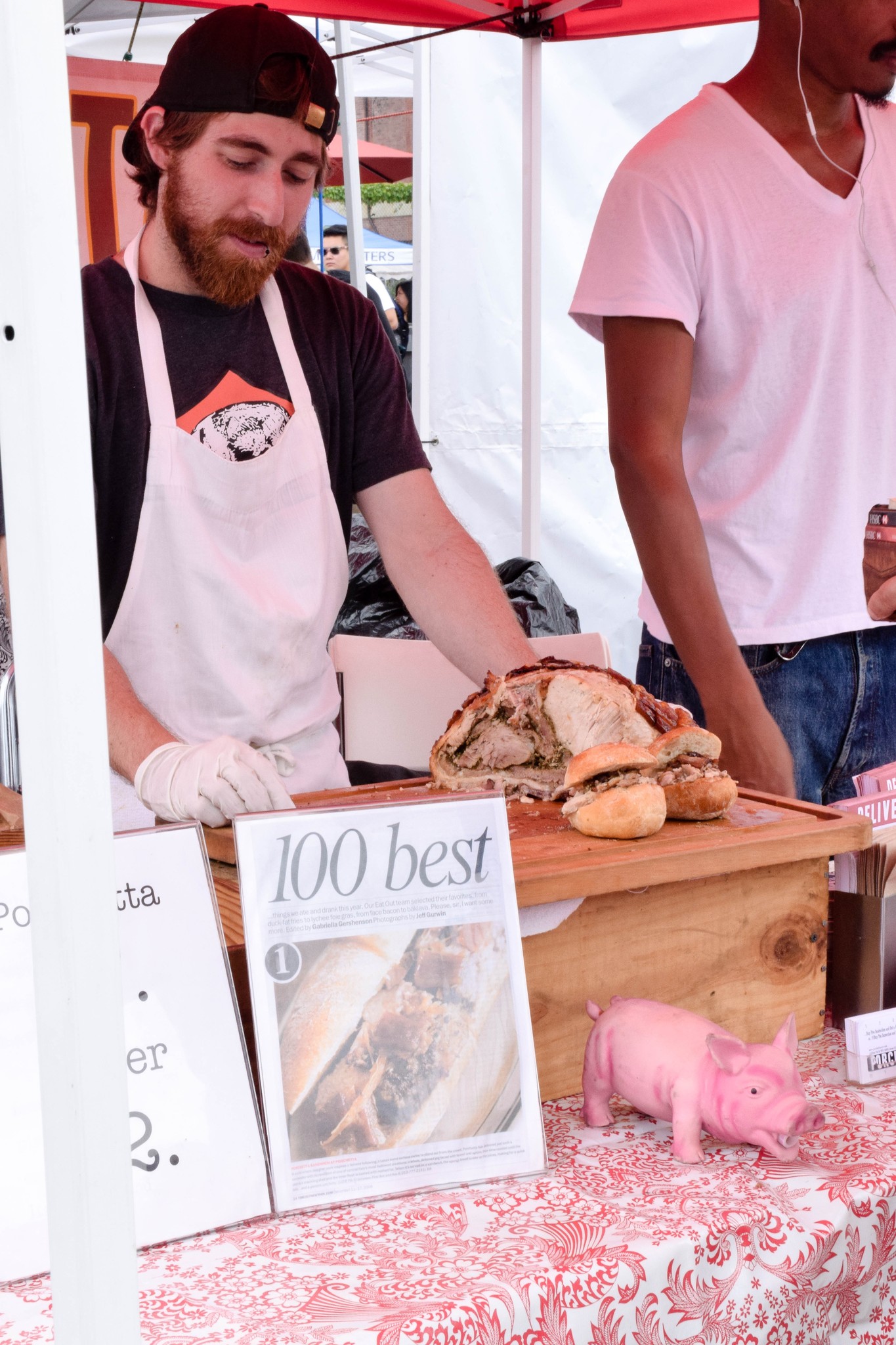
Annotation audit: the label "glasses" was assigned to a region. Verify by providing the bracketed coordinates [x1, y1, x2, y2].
[323, 247, 349, 256]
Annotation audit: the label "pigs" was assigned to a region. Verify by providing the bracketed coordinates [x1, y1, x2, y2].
[582, 992, 815, 1164]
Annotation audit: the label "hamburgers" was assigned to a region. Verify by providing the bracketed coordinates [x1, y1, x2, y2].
[648, 725, 738, 820]
[564, 743, 667, 839]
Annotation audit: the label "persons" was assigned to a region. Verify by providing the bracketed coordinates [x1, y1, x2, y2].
[321, 222, 399, 330]
[282, 230, 403, 368]
[569, 0, 896, 805]
[394, 278, 413, 325]
[0, 3, 542, 828]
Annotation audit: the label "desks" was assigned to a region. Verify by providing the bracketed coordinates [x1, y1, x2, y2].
[0, 1025, 896, 1345]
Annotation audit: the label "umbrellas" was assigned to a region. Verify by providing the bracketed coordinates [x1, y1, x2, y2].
[314, 133, 413, 186]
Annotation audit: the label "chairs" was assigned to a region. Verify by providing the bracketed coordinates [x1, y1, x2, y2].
[326, 630, 612, 776]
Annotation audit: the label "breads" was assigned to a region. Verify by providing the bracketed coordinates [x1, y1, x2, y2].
[281, 922, 518, 1147]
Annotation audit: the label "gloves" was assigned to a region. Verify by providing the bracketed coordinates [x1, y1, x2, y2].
[134, 738, 299, 826]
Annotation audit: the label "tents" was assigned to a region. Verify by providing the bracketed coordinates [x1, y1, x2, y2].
[301, 196, 416, 280]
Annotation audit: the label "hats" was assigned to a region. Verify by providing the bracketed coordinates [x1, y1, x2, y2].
[122, 4, 340, 165]
